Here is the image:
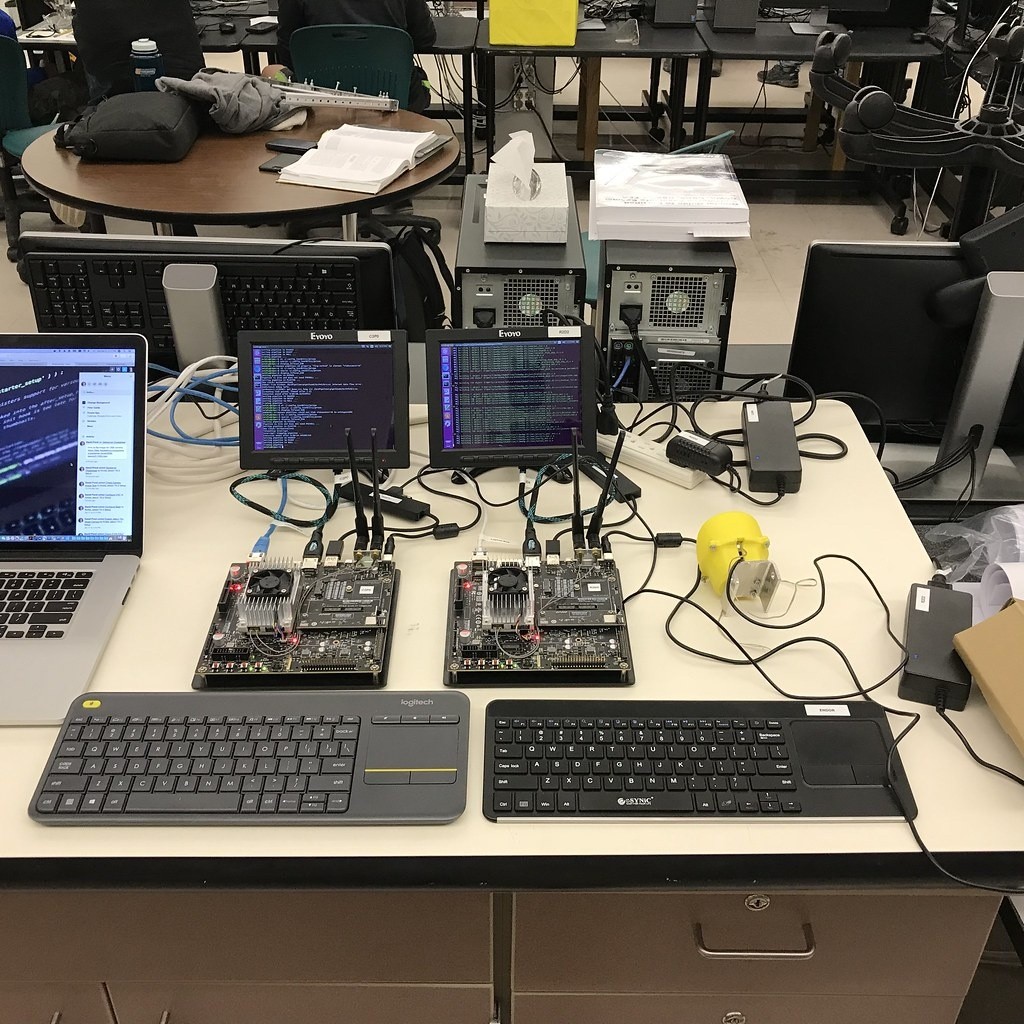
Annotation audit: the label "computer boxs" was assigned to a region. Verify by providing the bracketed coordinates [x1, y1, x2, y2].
[703, 0, 759, 34]
[452, 173, 587, 331]
[642, 0, 699, 30]
[593, 236, 738, 403]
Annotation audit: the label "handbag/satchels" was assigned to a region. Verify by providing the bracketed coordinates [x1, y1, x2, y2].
[52, 91, 202, 161]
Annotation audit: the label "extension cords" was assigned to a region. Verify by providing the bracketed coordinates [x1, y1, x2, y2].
[594, 424, 707, 490]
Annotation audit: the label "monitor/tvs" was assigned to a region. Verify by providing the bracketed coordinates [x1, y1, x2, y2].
[760, 0, 891, 36]
[424, 329, 597, 467]
[237, 329, 409, 469]
[780, 241, 1024, 448]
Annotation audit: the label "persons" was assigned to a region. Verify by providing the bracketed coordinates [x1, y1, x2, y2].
[0, 9, 57, 126]
[276, 0, 437, 215]
[72, 0, 206, 103]
[663, 58, 723, 77]
[757, 59, 804, 87]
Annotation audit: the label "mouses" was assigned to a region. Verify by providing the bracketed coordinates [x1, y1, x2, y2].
[219, 20, 235, 33]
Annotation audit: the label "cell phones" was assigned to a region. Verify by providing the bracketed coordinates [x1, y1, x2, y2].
[245, 22, 278, 34]
[265, 139, 317, 154]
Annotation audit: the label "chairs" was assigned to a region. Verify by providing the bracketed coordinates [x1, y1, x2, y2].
[0, 34, 91, 262]
[285, 25, 443, 245]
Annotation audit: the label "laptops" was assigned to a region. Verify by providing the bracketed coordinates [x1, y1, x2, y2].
[0, 332, 148, 726]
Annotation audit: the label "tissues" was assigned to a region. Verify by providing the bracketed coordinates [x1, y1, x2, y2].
[484, 131, 569, 243]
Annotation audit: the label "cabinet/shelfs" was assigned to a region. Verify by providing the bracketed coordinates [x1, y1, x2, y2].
[0, 399, 1024, 1024]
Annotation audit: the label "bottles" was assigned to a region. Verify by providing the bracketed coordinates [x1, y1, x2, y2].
[128, 38, 165, 93]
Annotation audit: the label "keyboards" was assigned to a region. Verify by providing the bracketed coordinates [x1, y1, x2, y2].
[482, 699, 917, 824]
[27, 250, 360, 404]
[196, 24, 206, 35]
[26, 690, 470, 827]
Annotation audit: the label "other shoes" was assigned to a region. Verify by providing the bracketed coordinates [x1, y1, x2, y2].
[663, 57, 673, 73]
[712, 57, 723, 77]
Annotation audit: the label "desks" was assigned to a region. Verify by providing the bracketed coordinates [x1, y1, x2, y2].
[0, 0, 947, 264]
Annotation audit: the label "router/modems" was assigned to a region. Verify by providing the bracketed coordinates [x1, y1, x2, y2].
[443, 426, 637, 689]
[191, 427, 402, 689]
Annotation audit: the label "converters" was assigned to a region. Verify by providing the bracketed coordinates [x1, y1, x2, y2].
[741, 401, 802, 494]
[898, 583, 973, 712]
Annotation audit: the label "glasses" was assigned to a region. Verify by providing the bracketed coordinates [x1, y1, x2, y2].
[25, 25, 59, 38]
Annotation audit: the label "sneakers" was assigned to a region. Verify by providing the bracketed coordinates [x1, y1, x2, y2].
[758, 64, 800, 88]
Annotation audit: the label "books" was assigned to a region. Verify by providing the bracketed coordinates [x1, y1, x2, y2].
[274, 123, 443, 195]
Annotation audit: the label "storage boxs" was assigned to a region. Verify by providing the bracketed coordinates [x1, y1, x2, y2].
[489, 0, 578, 46]
